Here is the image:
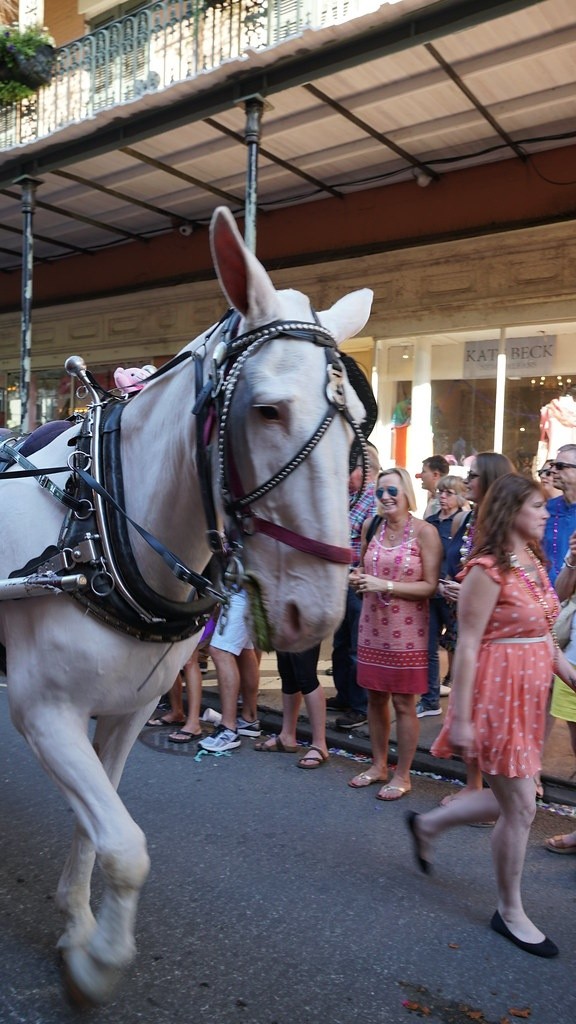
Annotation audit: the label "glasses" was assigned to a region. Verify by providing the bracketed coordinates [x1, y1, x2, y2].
[550, 462, 576, 470]
[466, 471, 480, 481]
[538, 469, 550, 476]
[436, 489, 457, 496]
[376, 486, 398, 499]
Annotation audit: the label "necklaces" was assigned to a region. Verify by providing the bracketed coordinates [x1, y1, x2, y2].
[509, 545, 559, 679]
[460, 509, 476, 565]
[372, 512, 414, 606]
[553, 497, 563, 574]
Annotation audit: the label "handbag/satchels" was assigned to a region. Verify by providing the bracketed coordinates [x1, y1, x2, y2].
[550, 596, 576, 649]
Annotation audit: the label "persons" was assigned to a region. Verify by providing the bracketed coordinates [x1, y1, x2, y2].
[415, 453, 518, 718]
[145, 587, 263, 753]
[404, 472, 576, 959]
[536, 444, 575, 854]
[348, 468, 443, 801]
[536, 397, 576, 482]
[254, 641, 329, 769]
[325, 440, 384, 728]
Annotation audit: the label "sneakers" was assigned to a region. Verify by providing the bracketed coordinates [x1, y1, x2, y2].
[336, 709, 368, 729]
[198, 724, 241, 751]
[214, 715, 262, 737]
[415, 700, 442, 718]
[325, 696, 352, 711]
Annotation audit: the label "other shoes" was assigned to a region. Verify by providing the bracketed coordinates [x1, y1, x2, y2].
[439, 680, 454, 695]
[406, 810, 430, 874]
[491, 910, 558, 957]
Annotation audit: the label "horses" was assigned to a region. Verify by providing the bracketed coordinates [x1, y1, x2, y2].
[0, 205, 373, 1017]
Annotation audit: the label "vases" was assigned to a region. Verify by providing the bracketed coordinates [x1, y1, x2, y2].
[0, 44, 54, 89]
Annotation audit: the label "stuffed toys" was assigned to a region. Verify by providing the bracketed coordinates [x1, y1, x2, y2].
[114, 365, 158, 396]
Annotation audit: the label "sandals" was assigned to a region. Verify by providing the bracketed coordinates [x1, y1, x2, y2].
[545, 835, 576, 854]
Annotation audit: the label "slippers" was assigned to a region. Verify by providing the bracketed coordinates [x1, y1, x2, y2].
[168, 730, 202, 743]
[145, 717, 185, 727]
[254, 735, 298, 752]
[348, 772, 388, 788]
[297, 747, 329, 768]
[439, 794, 458, 809]
[376, 785, 411, 800]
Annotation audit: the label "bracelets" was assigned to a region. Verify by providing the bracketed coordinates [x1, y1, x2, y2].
[387, 580, 393, 594]
[564, 555, 576, 570]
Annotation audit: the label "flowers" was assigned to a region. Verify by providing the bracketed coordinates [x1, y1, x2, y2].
[0, 21, 61, 107]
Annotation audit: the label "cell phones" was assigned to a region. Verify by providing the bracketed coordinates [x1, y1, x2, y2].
[439, 579, 451, 585]
